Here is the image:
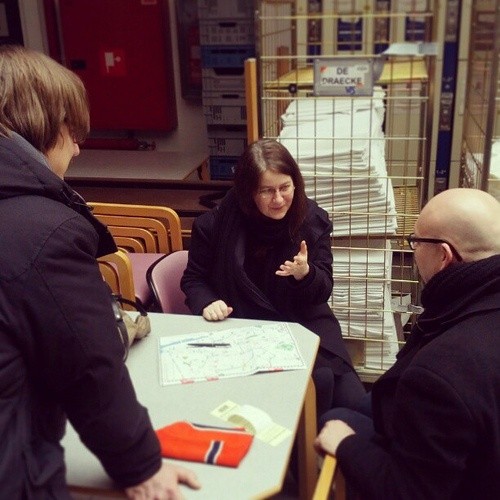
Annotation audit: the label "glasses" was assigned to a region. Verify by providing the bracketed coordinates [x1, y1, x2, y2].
[259, 182, 295, 198]
[406, 233, 463, 262]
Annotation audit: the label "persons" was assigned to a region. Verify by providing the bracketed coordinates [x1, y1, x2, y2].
[312, 188, 500, 500]
[179, 138, 368, 478]
[0, 46, 202, 500]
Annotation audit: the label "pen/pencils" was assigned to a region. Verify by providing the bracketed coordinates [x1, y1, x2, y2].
[188, 342, 231, 349]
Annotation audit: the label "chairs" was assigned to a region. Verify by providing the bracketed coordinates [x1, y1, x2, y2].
[146, 250, 194, 315]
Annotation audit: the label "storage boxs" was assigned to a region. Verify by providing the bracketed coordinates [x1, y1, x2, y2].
[196, 0, 256, 181]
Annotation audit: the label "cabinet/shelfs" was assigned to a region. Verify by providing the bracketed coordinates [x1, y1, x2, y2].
[0, 0, 177, 137]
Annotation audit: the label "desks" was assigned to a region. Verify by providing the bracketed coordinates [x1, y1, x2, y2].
[59, 311, 319, 500]
[63, 100, 213, 181]
